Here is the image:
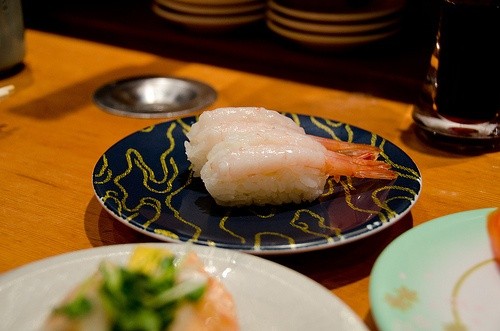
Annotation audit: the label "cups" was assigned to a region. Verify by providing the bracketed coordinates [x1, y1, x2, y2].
[0, 0, 26, 80]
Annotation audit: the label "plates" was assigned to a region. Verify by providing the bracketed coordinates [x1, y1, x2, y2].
[92, 73, 217, 118]
[368, 207, 500, 331]
[0, 243, 370, 331]
[151, 0, 265, 30]
[92, 111, 423, 255]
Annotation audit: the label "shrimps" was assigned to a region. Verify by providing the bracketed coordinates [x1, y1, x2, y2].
[185, 106, 398, 206]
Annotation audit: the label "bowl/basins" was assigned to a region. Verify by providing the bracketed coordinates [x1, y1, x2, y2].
[411, 106, 500, 157]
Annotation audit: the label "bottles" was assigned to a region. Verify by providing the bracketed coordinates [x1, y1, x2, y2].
[267, 0, 405, 50]
